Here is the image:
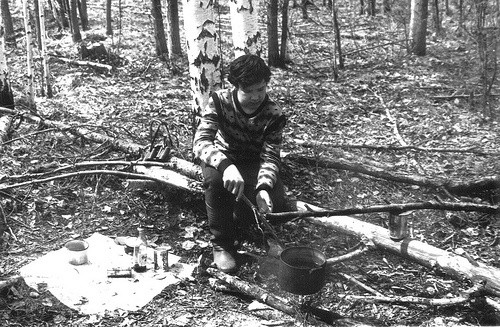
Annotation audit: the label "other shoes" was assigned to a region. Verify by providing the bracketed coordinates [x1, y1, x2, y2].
[211, 242, 237, 273]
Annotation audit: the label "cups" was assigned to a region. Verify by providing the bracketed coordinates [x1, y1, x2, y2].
[154, 247, 168, 272]
[66, 240, 90, 265]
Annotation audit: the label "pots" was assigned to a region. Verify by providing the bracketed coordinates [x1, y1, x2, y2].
[279, 245, 326, 296]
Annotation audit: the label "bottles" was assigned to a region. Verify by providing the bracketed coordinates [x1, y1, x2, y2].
[133, 226, 147, 273]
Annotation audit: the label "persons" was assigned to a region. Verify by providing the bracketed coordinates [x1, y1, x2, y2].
[191, 53, 286, 273]
